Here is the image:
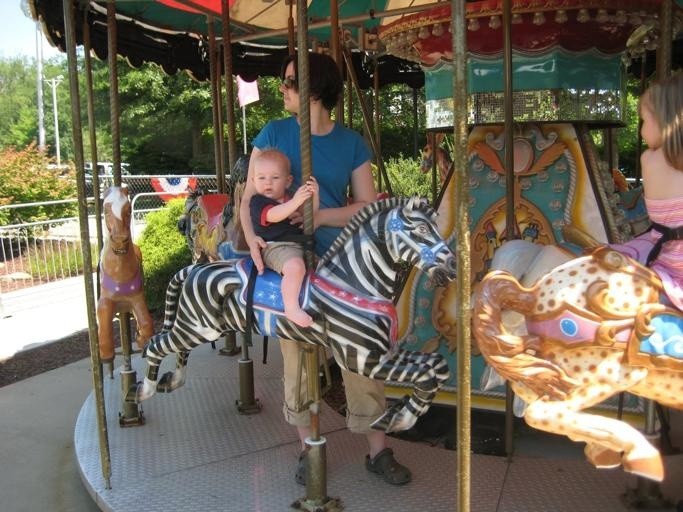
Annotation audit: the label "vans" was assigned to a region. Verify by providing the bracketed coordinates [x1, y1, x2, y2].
[85, 161, 130, 177]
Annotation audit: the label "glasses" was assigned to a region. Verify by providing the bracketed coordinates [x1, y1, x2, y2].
[281, 77, 294, 89]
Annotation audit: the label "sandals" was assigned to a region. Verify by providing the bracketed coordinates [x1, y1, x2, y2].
[365, 449, 411, 485]
[294, 450, 308, 486]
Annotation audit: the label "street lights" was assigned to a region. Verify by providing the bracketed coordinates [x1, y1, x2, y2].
[44, 75, 65, 168]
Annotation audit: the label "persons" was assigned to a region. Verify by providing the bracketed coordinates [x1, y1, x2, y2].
[248, 151, 314, 327]
[636, 75, 683, 276]
[233, 51, 413, 488]
